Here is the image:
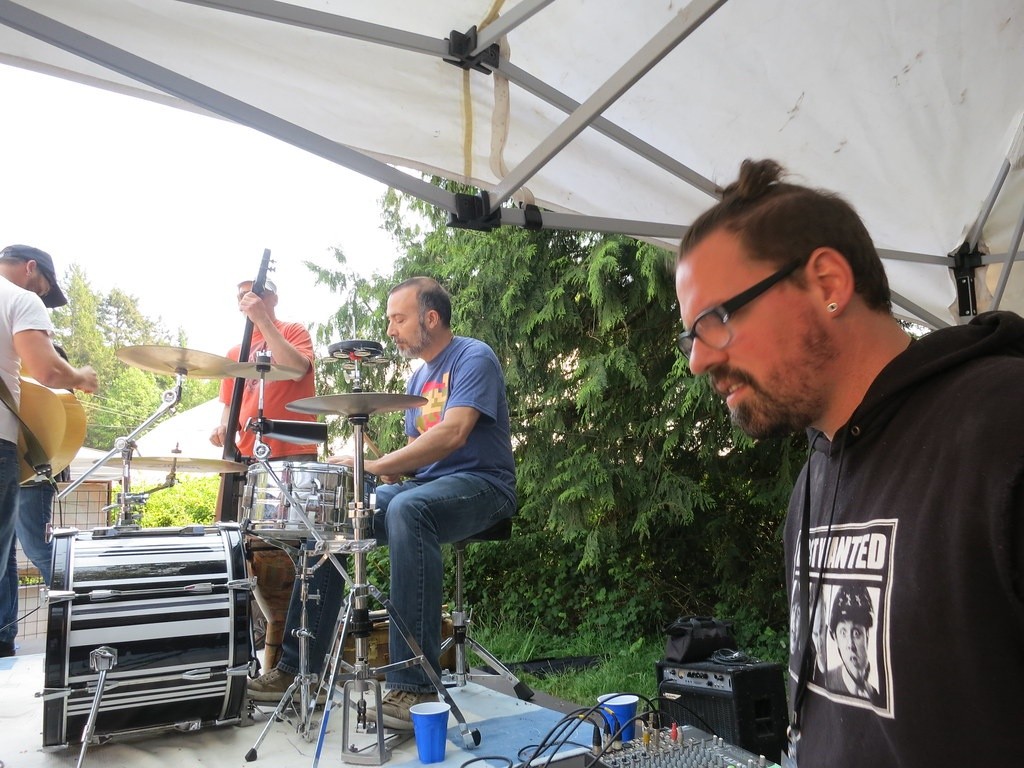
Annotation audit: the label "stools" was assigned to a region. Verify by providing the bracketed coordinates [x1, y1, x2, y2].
[373, 511, 535, 702]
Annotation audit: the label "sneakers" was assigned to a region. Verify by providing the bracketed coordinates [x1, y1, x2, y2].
[366, 690, 440, 731]
[246, 667, 326, 704]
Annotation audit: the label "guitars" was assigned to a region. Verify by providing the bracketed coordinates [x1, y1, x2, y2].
[17, 374, 89, 489]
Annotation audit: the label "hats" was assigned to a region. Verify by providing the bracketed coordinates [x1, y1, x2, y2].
[0, 244, 67, 308]
[238, 267, 277, 296]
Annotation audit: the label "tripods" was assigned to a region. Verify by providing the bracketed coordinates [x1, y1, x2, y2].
[245, 366, 481, 762]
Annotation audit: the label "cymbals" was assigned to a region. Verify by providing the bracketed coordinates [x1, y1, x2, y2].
[92, 456, 249, 474]
[114, 343, 239, 379]
[284, 393, 429, 418]
[222, 361, 305, 382]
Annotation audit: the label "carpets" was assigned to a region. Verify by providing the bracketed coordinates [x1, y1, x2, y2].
[0, 652, 604, 768]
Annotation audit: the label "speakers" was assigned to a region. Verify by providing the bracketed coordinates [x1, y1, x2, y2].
[656, 657, 785, 767]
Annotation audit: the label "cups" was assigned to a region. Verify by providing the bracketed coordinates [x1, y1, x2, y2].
[597, 692, 639, 742]
[409, 702, 452, 765]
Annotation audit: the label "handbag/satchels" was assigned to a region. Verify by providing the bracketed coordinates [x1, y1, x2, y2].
[663, 615, 738, 664]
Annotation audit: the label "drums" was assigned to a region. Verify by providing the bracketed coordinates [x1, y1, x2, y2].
[41, 523, 251, 752]
[242, 461, 379, 542]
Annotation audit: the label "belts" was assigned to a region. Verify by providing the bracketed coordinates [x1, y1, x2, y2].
[251, 454, 318, 462]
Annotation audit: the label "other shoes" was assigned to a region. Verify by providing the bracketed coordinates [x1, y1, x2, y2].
[0, 641, 15, 657]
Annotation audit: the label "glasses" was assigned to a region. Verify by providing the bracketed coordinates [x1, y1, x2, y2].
[676, 249, 819, 362]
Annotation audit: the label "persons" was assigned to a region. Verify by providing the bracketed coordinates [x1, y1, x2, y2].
[247, 274, 519, 733]
[0, 244, 99, 659]
[671, 154, 1024, 768]
[822, 584, 876, 699]
[210, 269, 320, 673]
